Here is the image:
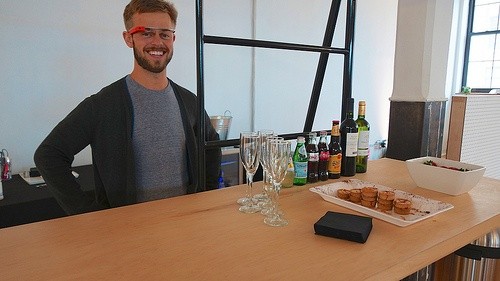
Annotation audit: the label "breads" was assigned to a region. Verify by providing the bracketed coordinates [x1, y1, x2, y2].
[337, 186, 412, 215]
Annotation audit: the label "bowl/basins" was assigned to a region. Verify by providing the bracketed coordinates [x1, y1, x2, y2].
[405, 156, 486, 196]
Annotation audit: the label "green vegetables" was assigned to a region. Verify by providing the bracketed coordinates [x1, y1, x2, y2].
[424, 160, 433, 165]
[458, 167, 469, 172]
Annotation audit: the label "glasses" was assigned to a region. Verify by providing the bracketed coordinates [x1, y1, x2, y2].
[129, 26, 175, 40]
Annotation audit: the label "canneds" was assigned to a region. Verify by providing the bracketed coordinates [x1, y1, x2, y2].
[0, 157, 11, 181]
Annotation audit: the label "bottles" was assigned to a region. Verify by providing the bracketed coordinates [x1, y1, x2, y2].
[305, 132, 319, 183]
[280, 141, 294, 188]
[339, 97, 359, 177]
[354, 100, 371, 173]
[317, 130, 330, 181]
[291, 137, 309, 185]
[328, 120, 343, 179]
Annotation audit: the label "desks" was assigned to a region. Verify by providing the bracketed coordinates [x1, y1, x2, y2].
[0, 157, 500, 281]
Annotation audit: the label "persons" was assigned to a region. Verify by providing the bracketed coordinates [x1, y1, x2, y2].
[34, 0, 222, 214]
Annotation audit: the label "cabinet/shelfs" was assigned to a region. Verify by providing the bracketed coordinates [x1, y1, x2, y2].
[0, 146, 241, 230]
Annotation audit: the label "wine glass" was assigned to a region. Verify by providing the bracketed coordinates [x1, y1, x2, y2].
[237, 129, 290, 227]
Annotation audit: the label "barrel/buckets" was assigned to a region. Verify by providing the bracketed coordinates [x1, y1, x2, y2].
[209, 110, 233, 150]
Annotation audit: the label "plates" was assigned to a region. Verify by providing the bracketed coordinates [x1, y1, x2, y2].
[309, 178, 454, 228]
